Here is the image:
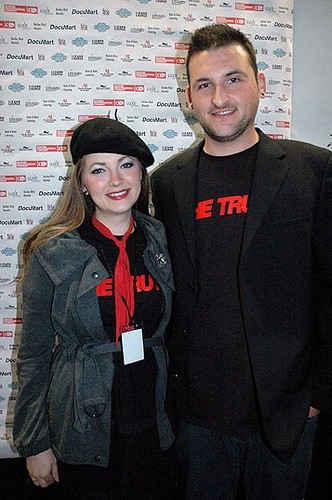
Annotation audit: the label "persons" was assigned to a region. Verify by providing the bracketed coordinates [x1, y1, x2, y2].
[12, 109, 177, 500]
[152, 25, 332, 500]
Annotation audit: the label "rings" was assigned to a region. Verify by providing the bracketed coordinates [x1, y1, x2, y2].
[33, 480, 38, 483]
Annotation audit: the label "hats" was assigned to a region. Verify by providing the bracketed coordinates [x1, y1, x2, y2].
[70, 118, 154, 169]
